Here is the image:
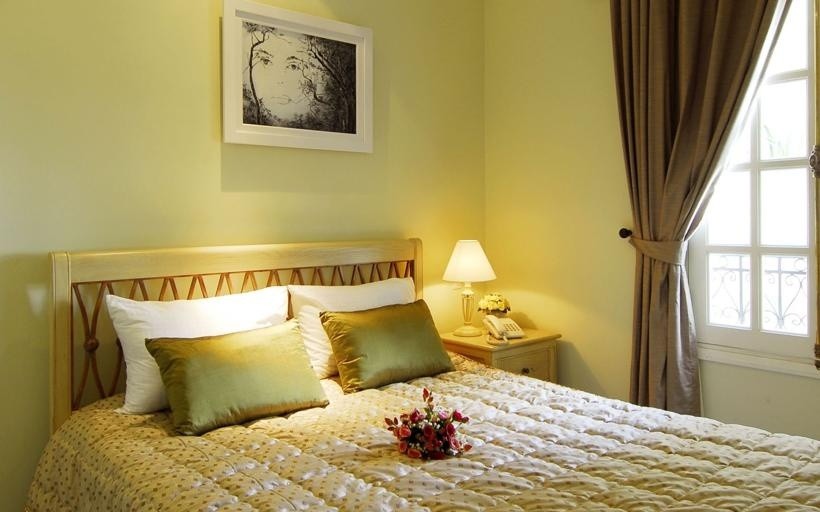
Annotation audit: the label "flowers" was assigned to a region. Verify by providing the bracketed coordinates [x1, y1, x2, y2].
[476, 293, 510, 313]
[385, 388, 472, 460]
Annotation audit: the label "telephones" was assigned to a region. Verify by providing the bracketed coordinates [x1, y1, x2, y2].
[483, 314, 524, 339]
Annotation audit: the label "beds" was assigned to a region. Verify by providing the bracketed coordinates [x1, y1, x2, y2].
[21, 238, 819, 512]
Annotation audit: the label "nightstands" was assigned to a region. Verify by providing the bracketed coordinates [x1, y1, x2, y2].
[439, 328, 562, 383]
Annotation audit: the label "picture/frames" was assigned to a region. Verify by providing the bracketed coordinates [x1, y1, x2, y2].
[223, 0, 375, 155]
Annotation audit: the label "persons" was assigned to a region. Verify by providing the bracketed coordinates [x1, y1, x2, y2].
[240, 21, 356, 135]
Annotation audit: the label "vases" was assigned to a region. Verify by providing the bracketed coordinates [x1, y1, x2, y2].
[486, 309, 506, 318]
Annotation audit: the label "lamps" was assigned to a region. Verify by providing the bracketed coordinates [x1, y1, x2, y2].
[442, 238, 498, 337]
